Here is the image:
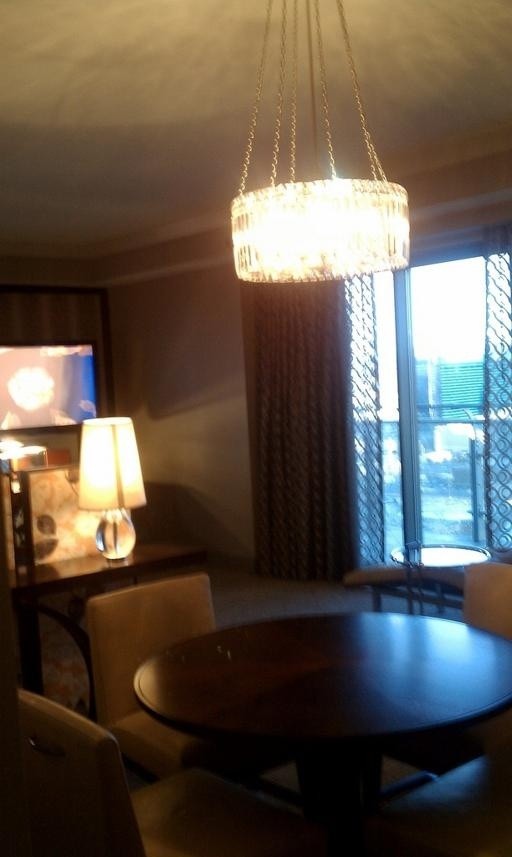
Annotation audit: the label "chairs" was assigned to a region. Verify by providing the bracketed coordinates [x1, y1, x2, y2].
[82, 572, 234, 776]
[2, 685, 329, 857]
[372, 561, 510, 857]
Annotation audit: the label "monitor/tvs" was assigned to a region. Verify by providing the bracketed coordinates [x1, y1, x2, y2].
[0, 341, 102, 436]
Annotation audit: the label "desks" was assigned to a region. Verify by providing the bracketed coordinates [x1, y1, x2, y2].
[132, 610, 508, 856]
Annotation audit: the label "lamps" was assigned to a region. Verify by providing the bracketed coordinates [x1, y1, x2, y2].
[0, 343, 148, 562]
[228, 0, 412, 286]
